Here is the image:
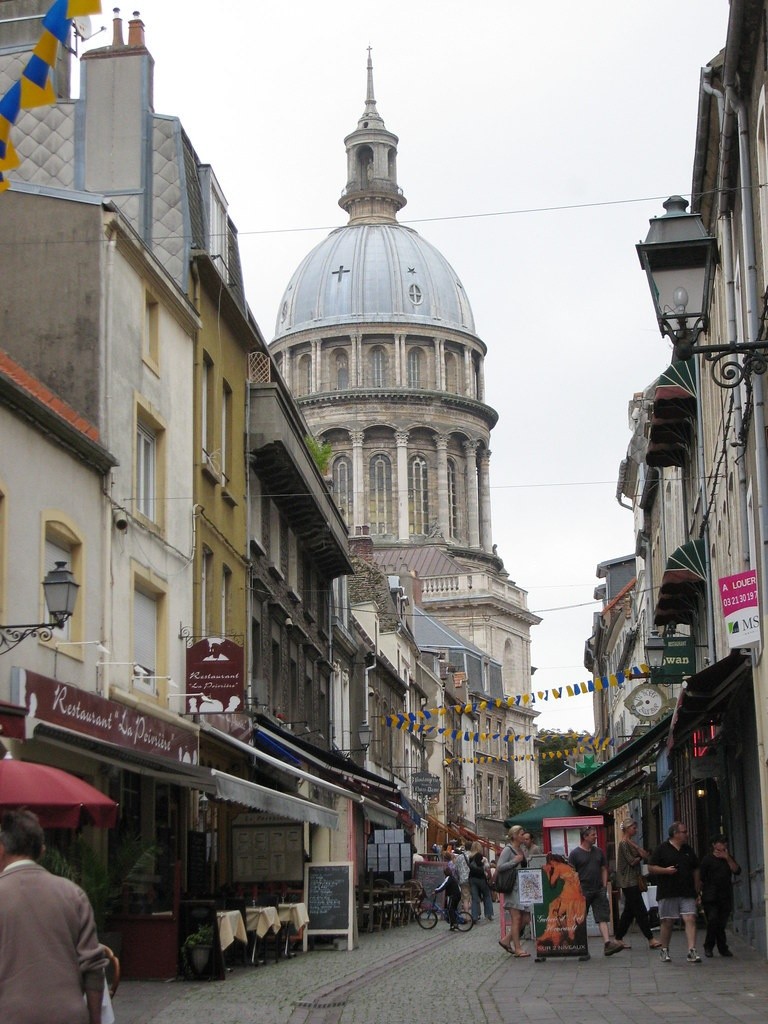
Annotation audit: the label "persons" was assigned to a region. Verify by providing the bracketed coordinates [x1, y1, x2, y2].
[521, 832, 543, 857]
[648, 821, 701, 962]
[569, 827, 623, 960]
[700, 832, 741, 957]
[0, 808, 110, 1024]
[614, 818, 661, 949]
[432, 867, 461, 930]
[496, 825, 532, 957]
[536, 852, 586, 954]
[412, 846, 425, 879]
[442, 838, 494, 923]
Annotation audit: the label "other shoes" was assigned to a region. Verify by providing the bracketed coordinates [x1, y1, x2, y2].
[720, 949, 733, 957]
[449, 927, 454, 931]
[649, 942, 662, 948]
[705, 948, 713, 957]
[464, 919, 471, 922]
[624, 945, 632, 949]
[485, 914, 493, 921]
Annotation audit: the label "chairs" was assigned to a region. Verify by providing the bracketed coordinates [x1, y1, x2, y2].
[374, 878, 392, 887]
[399, 879, 424, 923]
[221, 897, 260, 965]
[255, 895, 282, 957]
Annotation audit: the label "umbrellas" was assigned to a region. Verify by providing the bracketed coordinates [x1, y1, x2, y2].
[0, 759, 119, 830]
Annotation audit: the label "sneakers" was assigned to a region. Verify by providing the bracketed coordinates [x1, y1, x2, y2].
[687, 947, 701, 962]
[660, 947, 671, 961]
[604, 941, 623, 956]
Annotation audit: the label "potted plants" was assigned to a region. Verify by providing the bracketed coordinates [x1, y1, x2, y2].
[178, 924, 215, 980]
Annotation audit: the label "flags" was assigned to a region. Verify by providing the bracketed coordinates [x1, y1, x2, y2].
[382, 663, 648, 763]
[0, 0, 102, 192]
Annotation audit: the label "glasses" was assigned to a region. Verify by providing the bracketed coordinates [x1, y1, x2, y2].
[582, 826, 591, 835]
[678, 831, 687, 834]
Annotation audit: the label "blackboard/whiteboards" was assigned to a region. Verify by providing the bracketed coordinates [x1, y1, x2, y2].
[303, 861, 357, 934]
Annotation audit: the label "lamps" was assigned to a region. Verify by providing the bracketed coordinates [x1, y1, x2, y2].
[334, 719, 374, 763]
[279, 718, 313, 734]
[368, 687, 374, 696]
[644, 628, 667, 680]
[284, 618, 293, 629]
[167, 692, 214, 703]
[0, 556, 83, 657]
[637, 194, 768, 387]
[114, 508, 128, 530]
[133, 676, 179, 688]
[95, 661, 149, 676]
[54, 640, 110, 654]
[298, 728, 326, 740]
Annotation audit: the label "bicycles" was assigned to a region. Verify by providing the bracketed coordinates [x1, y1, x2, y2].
[417, 889, 474, 932]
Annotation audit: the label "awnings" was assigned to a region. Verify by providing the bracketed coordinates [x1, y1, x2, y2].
[665, 654, 752, 750]
[210, 770, 340, 832]
[262, 718, 398, 790]
[429, 814, 503, 854]
[646, 443, 686, 468]
[657, 592, 697, 610]
[653, 358, 696, 418]
[650, 419, 690, 445]
[660, 537, 706, 593]
[571, 713, 673, 803]
[653, 609, 693, 627]
[597, 784, 640, 812]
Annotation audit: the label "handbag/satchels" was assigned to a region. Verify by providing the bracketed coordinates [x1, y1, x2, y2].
[638, 875, 649, 891]
[490, 868, 516, 892]
[470, 860, 484, 875]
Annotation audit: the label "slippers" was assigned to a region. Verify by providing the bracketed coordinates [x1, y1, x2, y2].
[514, 952, 531, 957]
[499, 941, 514, 954]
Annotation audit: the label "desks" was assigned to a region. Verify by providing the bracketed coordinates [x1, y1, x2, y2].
[278, 903, 311, 958]
[356, 886, 425, 930]
[248, 906, 281, 964]
[152, 910, 248, 972]
